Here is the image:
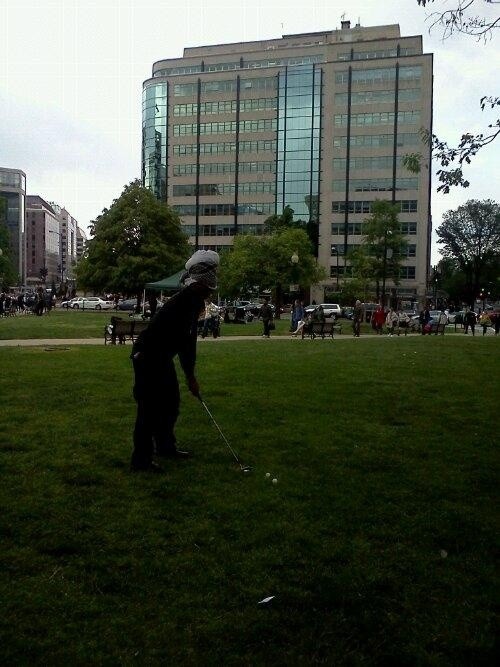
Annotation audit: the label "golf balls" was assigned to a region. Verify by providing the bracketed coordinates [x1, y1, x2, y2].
[266, 473, 271, 477]
[272, 479, 277, 484]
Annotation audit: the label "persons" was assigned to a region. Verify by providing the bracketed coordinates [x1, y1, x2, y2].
[372, 304, 447, 336]
[109, 289, 165, 321]
[258, 300, 273, 337]
[462, 306, 499, 336]
[2, 288, 56, 317]
[351, 300, 365, 337]
[129, 249, 220, 475]
[289, 299, 313, 337]
[199, 297, 220, 338]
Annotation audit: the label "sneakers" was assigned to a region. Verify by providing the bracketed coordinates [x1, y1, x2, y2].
[139, 461, 166, 473]
[172, 451, 192, 458]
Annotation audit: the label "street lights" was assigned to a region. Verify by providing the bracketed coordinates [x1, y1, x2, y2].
[432, 264, 439, 309]
[49, 230, 64, 297]
[289, 251, 299, 332]
[331, 246, 339, 303]
[375, 226, 392, 329]
[478, 288, 491, 312]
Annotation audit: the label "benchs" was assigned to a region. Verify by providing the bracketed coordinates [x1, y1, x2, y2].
[105, 320, 148, 343]
[196, 318, 221, 338]
[324, 318, 342, 334]
[424, 323, 448, 335]
[302, 322, 334, 340]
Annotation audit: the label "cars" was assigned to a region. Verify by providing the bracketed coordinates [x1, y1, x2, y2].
[304, 302, 380, 320]
[60, 293, 173, 310]
[399, 300, 493, 325]
[216, 299, 293, 314]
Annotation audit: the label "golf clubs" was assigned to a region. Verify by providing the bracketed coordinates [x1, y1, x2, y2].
[198, 396, 254, 477]
